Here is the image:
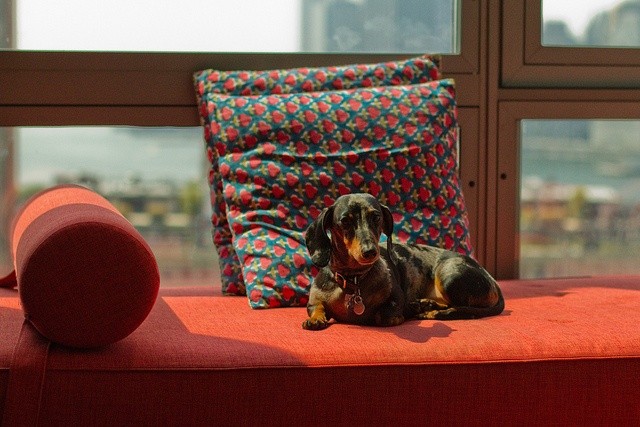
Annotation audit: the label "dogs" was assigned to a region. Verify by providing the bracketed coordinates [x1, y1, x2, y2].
[301, 193, 505, 332]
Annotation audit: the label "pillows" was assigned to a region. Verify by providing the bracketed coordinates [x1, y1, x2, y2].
[206, 78, 478, 312]
[193, 52, 443, 296]
[11, 183, 160, 349]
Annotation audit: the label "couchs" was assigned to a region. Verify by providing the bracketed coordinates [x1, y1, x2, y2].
[0, 269, 640, 427]
